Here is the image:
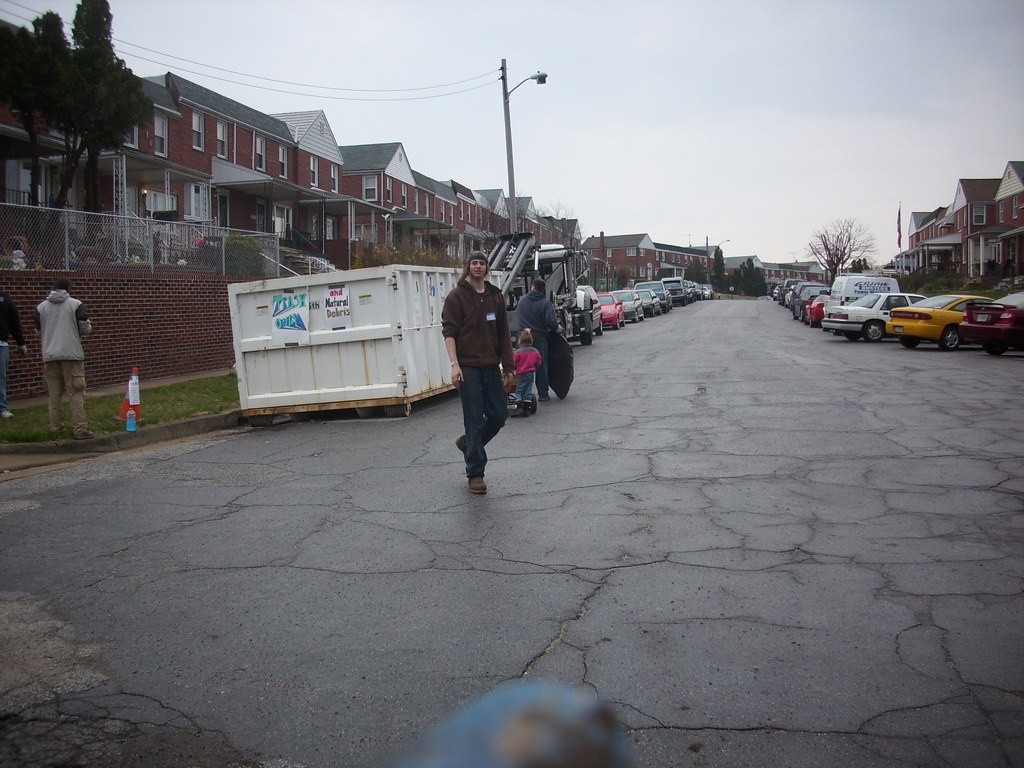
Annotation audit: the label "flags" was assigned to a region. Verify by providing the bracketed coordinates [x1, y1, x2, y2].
[897, 209, 901, 248]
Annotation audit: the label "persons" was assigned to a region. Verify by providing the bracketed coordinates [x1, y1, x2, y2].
[513, 328, 542, 403]
[515, 278, 564, 401]
[441, 252, 514, 493]
[0, 288, 27, 418]
[33, 277, 96, 439]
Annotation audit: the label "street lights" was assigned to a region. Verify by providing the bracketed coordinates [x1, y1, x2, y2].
[498, 56, 548, 235]
[706, 235, 730, 284]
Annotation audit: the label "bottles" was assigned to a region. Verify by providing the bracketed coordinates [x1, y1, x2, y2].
[125, 407, 137, 432]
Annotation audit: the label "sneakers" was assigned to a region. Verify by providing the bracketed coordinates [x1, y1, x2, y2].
[467, 476, 486, 493]
[455, 435, 467, 450]
[1, 410, 14, 418]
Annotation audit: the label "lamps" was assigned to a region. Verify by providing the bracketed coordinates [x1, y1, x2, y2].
[141, 190, 148, 197]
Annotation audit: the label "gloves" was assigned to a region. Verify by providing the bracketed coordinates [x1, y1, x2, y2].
[16, 345, 27, 355]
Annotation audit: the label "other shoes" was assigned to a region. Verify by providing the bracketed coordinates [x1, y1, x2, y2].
[538, 393, 550, 400]
[50, 422, 66, 432]
[515, 398, 523, 403]
[524, 397, 532, 403]
[74, 430, 94, 439]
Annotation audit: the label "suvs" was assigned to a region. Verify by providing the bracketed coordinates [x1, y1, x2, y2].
[574, 285, 604, 346]
[635, 281, 673, 314]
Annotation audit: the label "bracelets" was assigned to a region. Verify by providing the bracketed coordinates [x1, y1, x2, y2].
[450, 361, 458, 367]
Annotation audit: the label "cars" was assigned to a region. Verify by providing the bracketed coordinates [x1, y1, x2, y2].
[661, 277, 721, 307]
[793, 282, 831, 322]
[821, 291, 928, 344]
[595, 292, 626, 330]
[958, 292, 1024, 355]
[772, 279, 829, 312]
[804, 293, 831, 328]
[609, 289, 645, 323]
[885, 295, 994, 352]
[635, 289, 662, 318]
[755, 296, 774, 302]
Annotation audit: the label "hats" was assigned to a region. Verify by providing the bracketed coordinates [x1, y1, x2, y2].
[465, 251, 488, 264]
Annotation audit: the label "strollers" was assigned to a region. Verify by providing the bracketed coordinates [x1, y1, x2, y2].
[499, 365, 537, 415]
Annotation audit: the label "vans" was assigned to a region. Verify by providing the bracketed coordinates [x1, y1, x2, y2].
[827, 273, 900, 309]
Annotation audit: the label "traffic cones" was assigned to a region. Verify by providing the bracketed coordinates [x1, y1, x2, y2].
[113, 366, 145, 424]
